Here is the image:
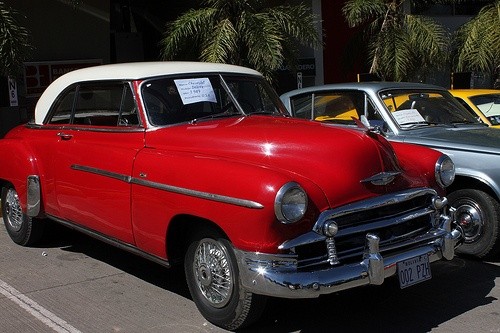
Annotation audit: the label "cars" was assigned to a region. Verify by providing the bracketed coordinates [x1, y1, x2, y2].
[314, 88, 499, 130]
[275, 80, 500, 259]
[0, 61, 466, 331]
